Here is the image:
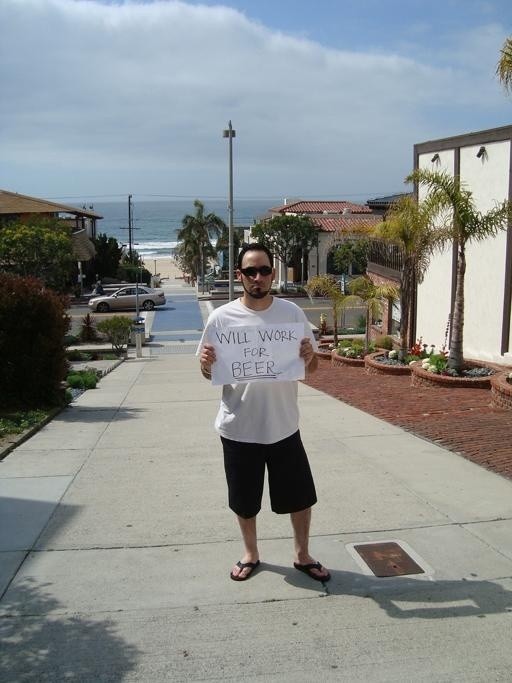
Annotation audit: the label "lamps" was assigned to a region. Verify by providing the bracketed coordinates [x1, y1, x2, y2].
[431, 154, 439, 162]
[477, 146, 486, 158]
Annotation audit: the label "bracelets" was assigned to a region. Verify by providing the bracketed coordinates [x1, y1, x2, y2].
[201, 365, 211, 375]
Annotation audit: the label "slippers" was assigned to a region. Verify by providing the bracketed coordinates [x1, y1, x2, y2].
[231, 559, 260, 581]
[293, 561, 330, 582]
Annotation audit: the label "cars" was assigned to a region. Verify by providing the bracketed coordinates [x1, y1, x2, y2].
[88, 285, 166, 312]
[280, 281, 297, 294]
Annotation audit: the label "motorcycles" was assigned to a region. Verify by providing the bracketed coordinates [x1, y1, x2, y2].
[88, 288, 112, 301]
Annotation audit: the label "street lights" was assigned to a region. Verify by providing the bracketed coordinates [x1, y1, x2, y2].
[222, 119, 235, 302]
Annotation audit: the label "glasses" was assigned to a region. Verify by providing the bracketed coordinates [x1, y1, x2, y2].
[239, 265, 273, 276]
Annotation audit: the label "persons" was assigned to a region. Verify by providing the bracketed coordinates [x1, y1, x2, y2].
[196, 243, 330, 581]
